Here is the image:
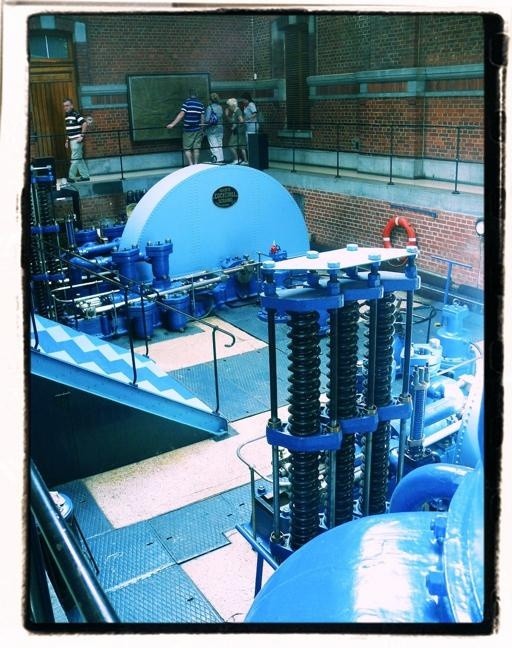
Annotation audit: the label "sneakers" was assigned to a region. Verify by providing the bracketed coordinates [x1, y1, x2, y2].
[240, 161, 249, 165]
[231, 158, 242, 165]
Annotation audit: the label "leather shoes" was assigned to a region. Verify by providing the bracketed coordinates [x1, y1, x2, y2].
[66, 177, 90, 184]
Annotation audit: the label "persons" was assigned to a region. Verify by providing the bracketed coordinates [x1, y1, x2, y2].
[166, 87, 206, 165]
[225, 97, 249, 165]
[62, 98, 90, 183]
[240, 93, 260, 136]
[203, 93, 224, 162]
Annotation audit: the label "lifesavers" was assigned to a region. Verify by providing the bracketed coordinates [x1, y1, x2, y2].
[383, 217, 416, 260]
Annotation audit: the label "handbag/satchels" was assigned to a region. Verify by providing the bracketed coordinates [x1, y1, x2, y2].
[205, 111, 219, 127]
[236, 123, 247, 134]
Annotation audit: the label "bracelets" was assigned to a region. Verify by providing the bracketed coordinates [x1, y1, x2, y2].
[81, 135, 86, 137]
